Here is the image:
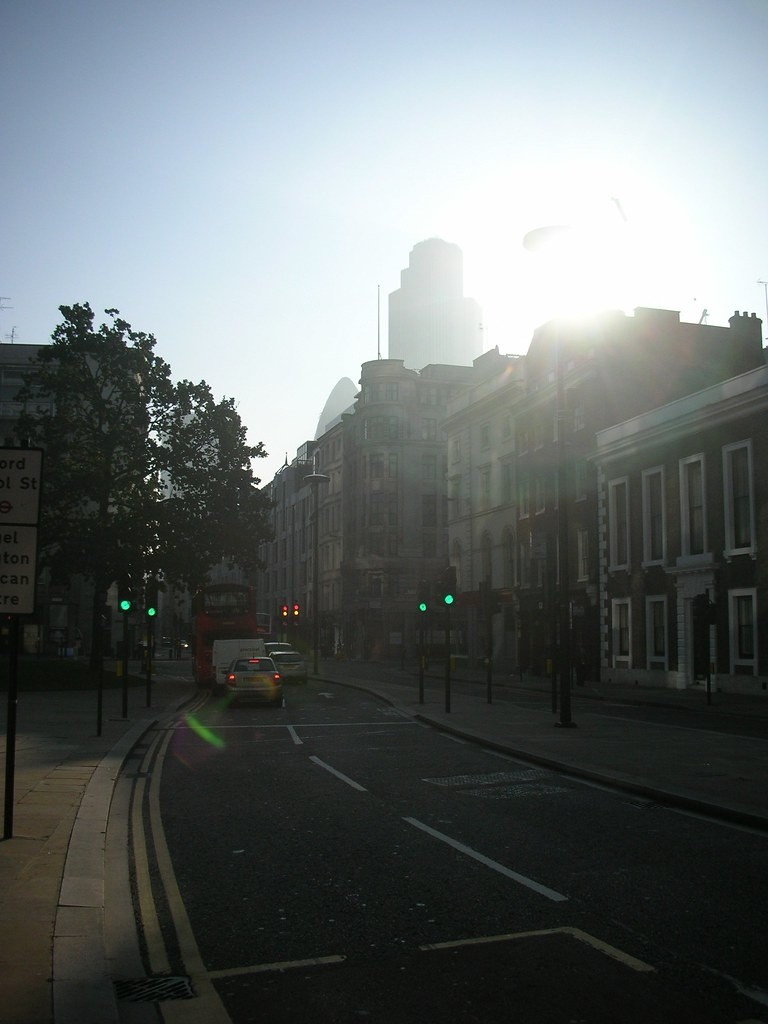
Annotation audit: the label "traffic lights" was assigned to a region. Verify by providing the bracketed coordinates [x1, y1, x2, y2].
[419, 578, 433, 617]
[692, 594, 710, 637]
[117, 571, 133, 614]
[294, 603, 304, 624]
[441, 566, 457, 607]
[282, 605, 290, 620]
[146, 580, 158, 620]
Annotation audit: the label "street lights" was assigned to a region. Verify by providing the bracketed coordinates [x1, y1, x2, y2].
[301, 473, 333, 677]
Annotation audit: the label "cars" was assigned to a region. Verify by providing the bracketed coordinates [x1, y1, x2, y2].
[265, 643, 294, 655]
[269, 650, 307, 683]
[163, 639, 170, 646]
[222, 657, 286, 708]
[180, 640, 188, 649]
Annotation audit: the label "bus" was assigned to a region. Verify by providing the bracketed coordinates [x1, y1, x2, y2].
[190, 584, 260, 671]
